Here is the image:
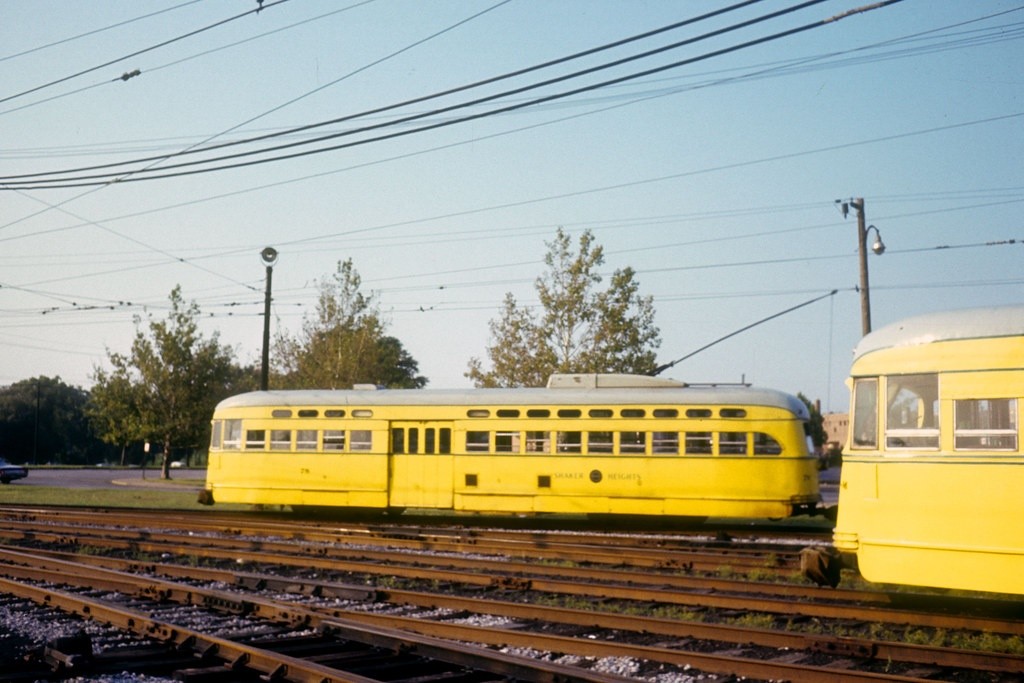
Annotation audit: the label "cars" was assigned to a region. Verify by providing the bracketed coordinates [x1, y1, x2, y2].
[0, 457, 29, 484]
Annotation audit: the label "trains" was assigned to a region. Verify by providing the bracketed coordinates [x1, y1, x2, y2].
[197, 373, 824, 528]
[832, 304, 1023, 600]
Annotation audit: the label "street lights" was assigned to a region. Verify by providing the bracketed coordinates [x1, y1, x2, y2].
[858, 224, 885, 337]
